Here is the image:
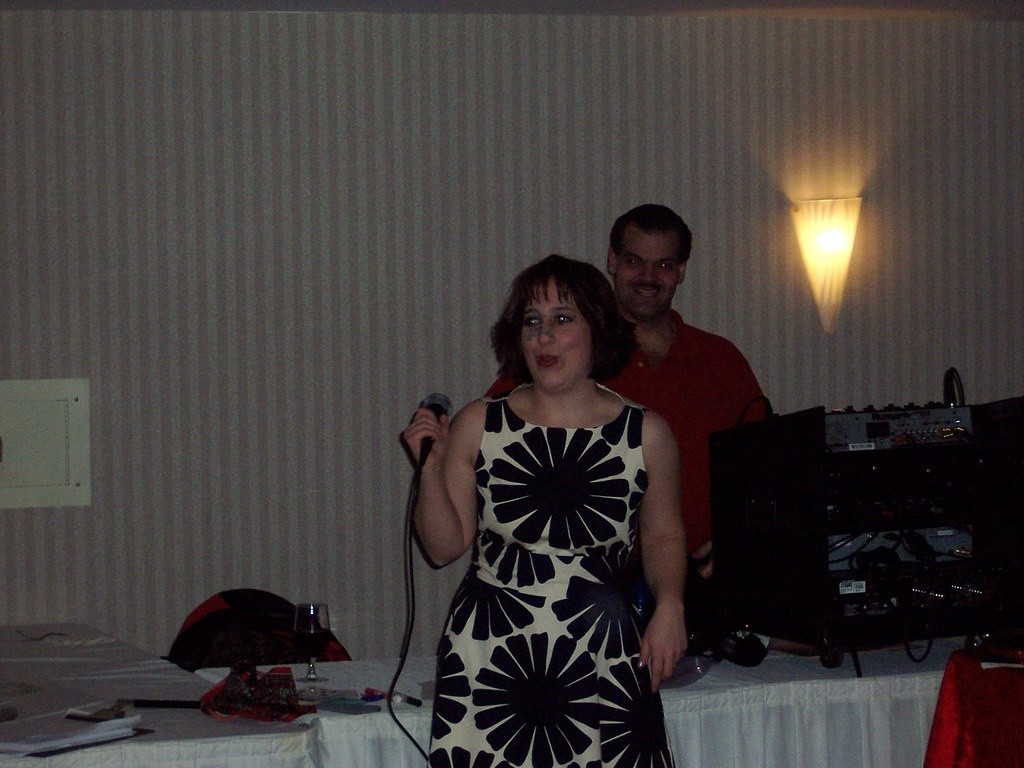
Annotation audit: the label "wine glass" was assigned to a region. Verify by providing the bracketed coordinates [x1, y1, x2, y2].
[294, 602, 331, 683]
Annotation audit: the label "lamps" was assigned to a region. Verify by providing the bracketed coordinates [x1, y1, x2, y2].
[790, 197, 864, 334]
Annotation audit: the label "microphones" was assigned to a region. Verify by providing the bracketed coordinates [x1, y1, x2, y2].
[417, 394, 453, 469]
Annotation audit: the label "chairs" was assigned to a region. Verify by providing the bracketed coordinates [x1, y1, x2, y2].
[166, 587, 351, 675]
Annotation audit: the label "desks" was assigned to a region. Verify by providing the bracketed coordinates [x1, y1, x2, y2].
[0, 621, 1022, 768]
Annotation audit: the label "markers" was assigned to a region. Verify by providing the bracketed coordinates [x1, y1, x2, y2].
[364, 687, 402, 703]
[391, 689, 422, 707]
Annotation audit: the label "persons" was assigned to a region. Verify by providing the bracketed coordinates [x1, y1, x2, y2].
[404, 254, 687, 768]
[488, 204, 776, 583]
